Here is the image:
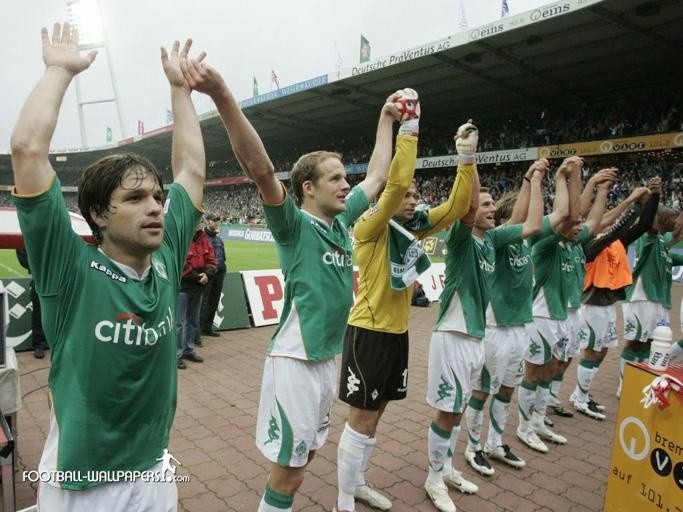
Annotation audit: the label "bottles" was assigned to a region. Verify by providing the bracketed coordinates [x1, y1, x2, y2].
[648, 325, 673, 372]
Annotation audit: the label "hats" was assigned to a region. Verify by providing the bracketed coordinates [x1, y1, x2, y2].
[206, 214, 220, 221]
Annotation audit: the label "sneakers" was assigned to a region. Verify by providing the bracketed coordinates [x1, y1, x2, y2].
[332, 500, 350, 512]
[464, 444, 495, 476]
[34, 347, 43, 358]
[177, 331, 219, 368]
[355, 484, 392, 511]
[425, 478, 457, 512]
[443, 471, 479, 494]
[483, 441, 525, 469]
[517, 393, 606, 455]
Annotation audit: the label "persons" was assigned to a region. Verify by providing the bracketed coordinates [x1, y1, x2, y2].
[0, 22, 683, 512]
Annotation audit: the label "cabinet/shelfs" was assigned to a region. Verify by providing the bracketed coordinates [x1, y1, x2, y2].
[601, 358, 683, 510]
